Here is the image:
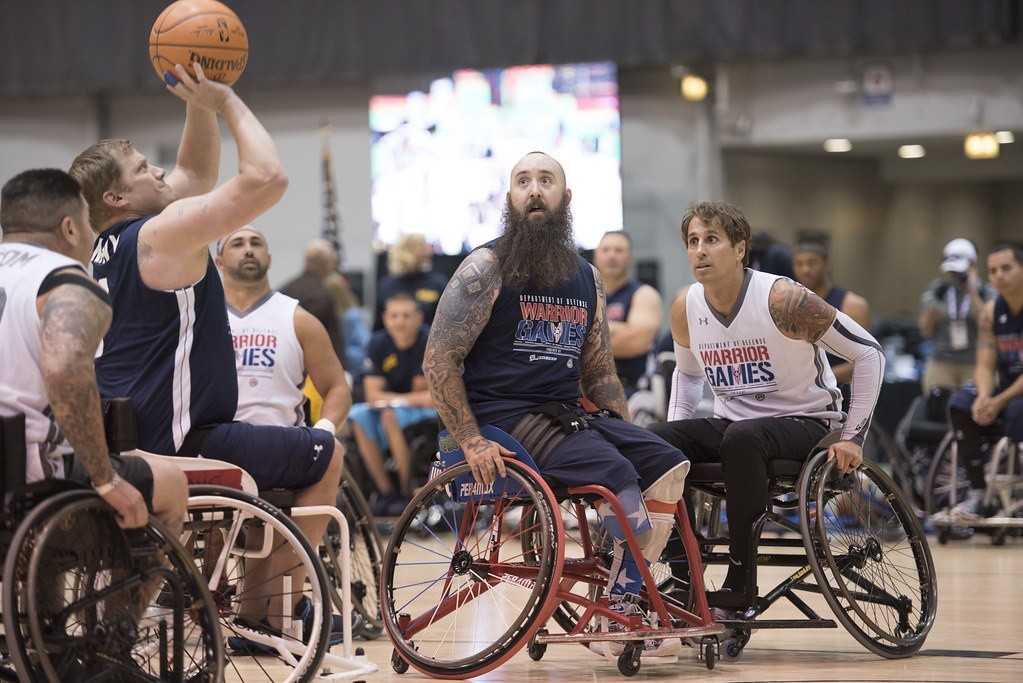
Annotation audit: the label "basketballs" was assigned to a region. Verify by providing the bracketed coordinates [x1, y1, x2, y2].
[148, 0, 250, 87]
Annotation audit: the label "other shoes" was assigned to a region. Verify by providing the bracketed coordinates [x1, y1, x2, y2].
[708, 588, 769, 610]
[387, 494, 412, 517]
[949, 483, 998, 519]
[81, 639, 160, 683]
[371, 486, 396, 515]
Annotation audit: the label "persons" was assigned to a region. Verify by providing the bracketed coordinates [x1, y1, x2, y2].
[0, 168, 188, 683]
[422, 151, 691, 660]
[942, 241, 1023, 519]
[646, 199, 887, 636]
[215, 224, 451, 652]
[69, 60, 365, 657]
[789, 227, 874, 526]
[920, 239, 997, 398]
[594, 231, 666, 428]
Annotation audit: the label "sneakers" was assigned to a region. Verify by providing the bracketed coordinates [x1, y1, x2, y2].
[228, 615, 268, 650]
[589, 591, 682, 657]
[254, 597, 363, 652]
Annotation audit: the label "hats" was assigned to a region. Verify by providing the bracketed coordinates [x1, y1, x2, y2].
[941, 238, 977, 272]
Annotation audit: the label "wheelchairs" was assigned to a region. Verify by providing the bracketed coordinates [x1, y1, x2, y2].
[0, 411, 226, 682]
[588, 429, 937, 661]
[845, 388, 1022, 548]
[73, 397, 379, 683]
[341, 418, 494, 535]
[379, 411, 725, 680]
[192, 465, 384, 640]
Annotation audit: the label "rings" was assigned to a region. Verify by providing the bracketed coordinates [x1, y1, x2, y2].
[849, 463, 855, 468]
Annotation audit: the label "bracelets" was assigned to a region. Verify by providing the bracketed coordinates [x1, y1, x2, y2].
[91, 471, 121, 495]
